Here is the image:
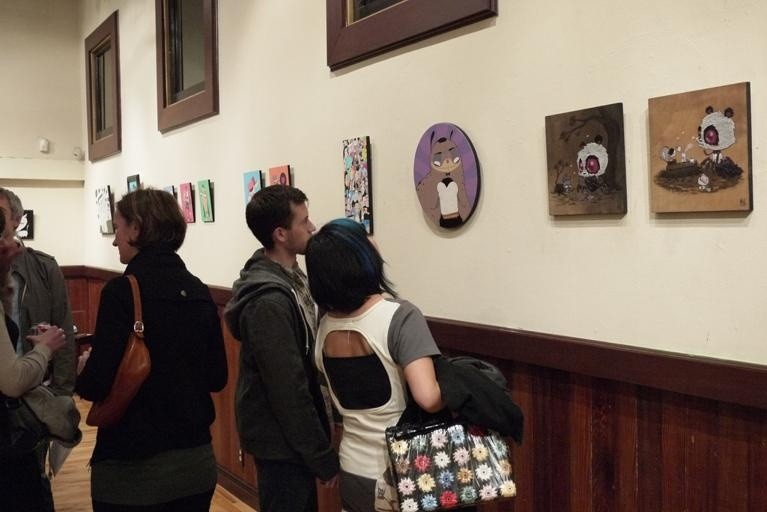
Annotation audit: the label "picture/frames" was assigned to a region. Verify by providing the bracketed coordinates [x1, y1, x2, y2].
[126, 173, 140, 192]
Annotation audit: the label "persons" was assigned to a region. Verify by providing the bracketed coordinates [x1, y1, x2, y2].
[221, 181, 334, 511]
[0, 206, 67, 512]
[72, 184, 229, 511]
[1, 184, 81, 512]
[304, 215, 446, 511]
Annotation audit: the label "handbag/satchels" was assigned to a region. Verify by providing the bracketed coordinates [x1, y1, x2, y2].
[384, 422, 518, 512]
[86, 334, 151, 427]
